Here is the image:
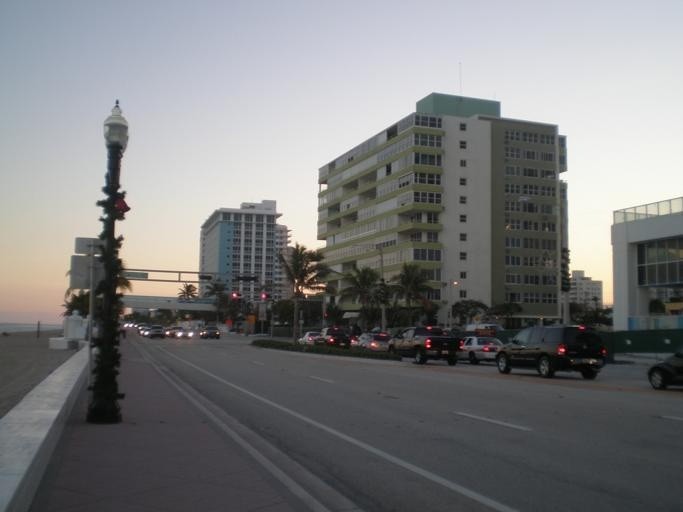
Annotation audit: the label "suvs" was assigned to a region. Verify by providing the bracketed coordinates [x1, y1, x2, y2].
[496, 324, 609, 381]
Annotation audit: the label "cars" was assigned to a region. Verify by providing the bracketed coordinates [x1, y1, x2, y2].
[648, 350, 683, 391]
[124, 322, 220, 341]
[299, 324, 506, 366]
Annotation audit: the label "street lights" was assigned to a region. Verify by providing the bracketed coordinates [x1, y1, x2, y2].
[88, 100, 128, 427]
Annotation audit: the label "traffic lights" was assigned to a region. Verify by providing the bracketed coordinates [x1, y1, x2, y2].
[232, 293, 238, 299]
[262, 293, 268, 299]
